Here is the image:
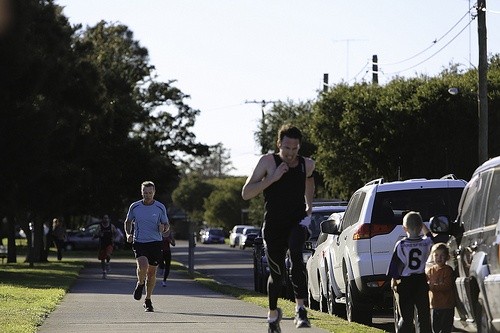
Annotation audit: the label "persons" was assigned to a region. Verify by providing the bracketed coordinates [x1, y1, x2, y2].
[92, 213, 175, 287]
[124, 181, 170, 312]
[386, 211, 458, 333]
[52, 218, 67, 260]
[241, 124, 315, 333]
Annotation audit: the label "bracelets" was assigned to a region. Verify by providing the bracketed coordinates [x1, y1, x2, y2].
[163, 224, 168, 233]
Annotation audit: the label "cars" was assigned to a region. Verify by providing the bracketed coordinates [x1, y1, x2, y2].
[63, 227, 100, 251]
[252, 172, 469, 327]
[239, 227, 260, 250]
[428, 155, 500, 333]
[201, 226, 225, 244]
[229, 224, 254, 248]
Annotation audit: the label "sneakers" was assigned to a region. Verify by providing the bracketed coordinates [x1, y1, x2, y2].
[133, 280, 145, 300]
[268, 307, 282, 333]
[142, 300, 153, 312]
[294, 306, 311, 329]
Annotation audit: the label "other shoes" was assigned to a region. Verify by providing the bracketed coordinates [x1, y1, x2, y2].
[106, 264, 111, 272]
[158, 267, 163, 275]
[103, 270, 107, 273]
[162, 282, 166, 287]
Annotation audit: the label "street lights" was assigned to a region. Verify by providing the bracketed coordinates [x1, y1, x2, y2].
[447, 87, 489, 167]
